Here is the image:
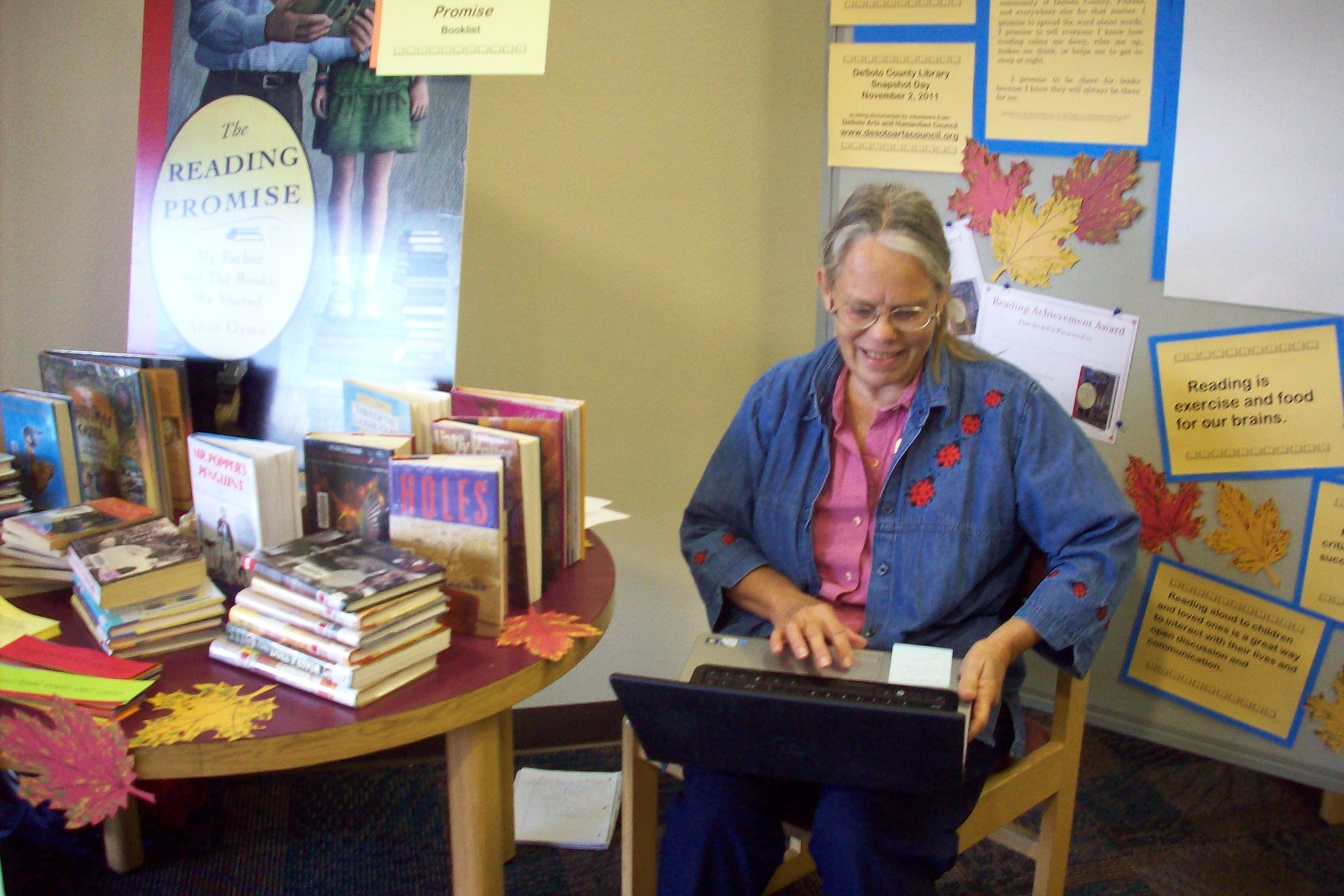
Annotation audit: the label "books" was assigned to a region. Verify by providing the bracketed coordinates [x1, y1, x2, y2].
[304, 375, 585, 637]
[207, 531, 451, 708]
[0, 351, 304, 729]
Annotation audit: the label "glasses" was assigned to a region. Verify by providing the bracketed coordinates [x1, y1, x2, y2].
[831, 294, 940, 330]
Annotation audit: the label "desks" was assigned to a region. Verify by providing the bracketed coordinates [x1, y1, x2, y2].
[0, 533, 617, 896]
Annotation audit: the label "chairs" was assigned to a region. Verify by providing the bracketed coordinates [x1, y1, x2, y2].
[618, 554, 1090, 896]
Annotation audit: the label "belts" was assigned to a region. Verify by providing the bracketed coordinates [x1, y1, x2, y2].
[209, 69, 300, 90]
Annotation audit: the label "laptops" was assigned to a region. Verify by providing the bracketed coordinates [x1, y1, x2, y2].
[608, 630, 972, 801]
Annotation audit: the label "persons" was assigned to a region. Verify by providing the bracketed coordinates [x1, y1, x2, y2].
[162, 416, 183, 452]
[81, 532, 157, 587]
[658, 184, 1139, 896]
[189, 5, 433, 270]
